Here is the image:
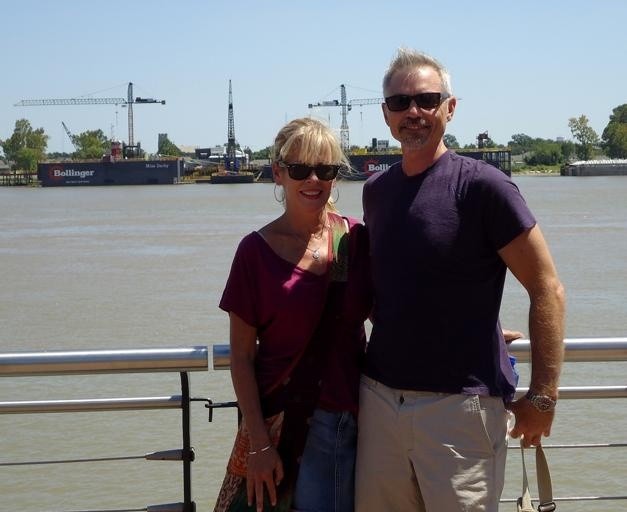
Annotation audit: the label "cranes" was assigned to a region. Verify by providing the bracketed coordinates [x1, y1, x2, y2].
[14, 83, 167, 157]
[307, 84, 462, 164]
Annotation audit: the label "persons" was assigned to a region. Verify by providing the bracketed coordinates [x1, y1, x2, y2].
[352, 45, 566, 511]
[210, 117, 527, 512]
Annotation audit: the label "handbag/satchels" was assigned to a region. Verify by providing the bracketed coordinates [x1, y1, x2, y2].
[213, 394, 313, 511]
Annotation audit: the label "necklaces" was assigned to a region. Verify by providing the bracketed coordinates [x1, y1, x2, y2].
[282, 214, 330, 260]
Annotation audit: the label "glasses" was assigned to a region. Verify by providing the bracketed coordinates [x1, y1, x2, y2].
[279, 161, 340, 181]
[385, 93, 450, 111]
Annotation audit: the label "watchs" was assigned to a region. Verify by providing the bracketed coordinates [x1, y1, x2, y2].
[524, 389, 557, 412]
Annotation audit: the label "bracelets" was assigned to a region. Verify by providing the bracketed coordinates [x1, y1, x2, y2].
[248, 442, 274, 455]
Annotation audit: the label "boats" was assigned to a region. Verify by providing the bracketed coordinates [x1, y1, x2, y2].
[211, 171, 254, 183]
